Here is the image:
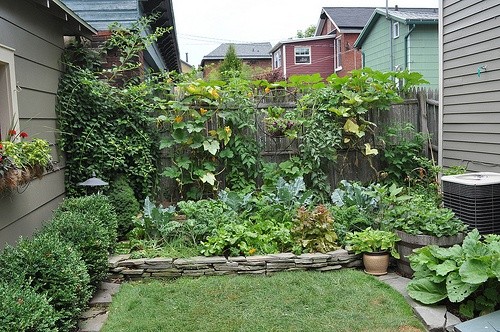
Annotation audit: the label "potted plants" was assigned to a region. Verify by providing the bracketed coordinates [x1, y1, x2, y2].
[352, 228, 401, 276]
[398, 195, 469, 278]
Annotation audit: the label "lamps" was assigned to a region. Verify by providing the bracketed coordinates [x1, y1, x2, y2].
[78, 172, 109, 185]
